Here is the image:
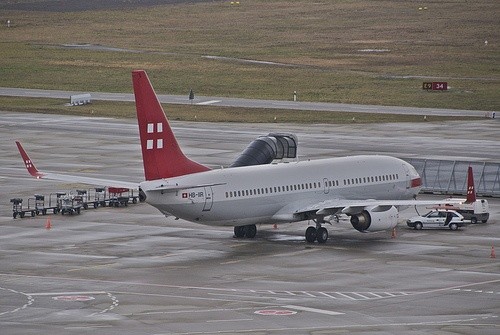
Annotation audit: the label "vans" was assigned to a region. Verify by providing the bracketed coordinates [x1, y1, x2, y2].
[436, 198, 490, 224]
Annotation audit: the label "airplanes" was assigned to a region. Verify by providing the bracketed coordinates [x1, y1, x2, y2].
[15, 69, 477, 245]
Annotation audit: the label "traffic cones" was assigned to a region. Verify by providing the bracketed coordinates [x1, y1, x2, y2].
[489, 241, 497, 259]
[391, 228, 396, 237]
[46, 216, 52, 229]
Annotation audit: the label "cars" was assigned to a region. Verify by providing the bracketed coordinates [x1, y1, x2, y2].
[406, 210, 464, 231]
